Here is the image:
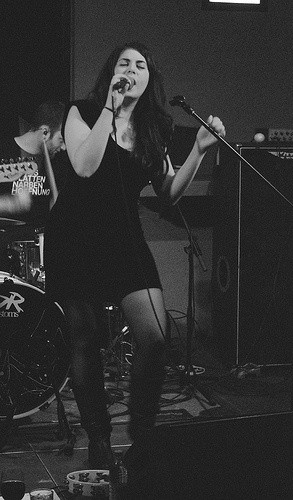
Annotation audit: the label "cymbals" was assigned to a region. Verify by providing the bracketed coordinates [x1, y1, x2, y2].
[0, 217, 26, 229]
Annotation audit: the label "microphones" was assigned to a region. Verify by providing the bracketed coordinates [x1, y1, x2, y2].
[111, 76, 135, 91]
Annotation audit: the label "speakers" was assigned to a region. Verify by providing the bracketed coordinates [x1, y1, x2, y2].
[110, 408, 293, 500]
[213, 139, 293, 370]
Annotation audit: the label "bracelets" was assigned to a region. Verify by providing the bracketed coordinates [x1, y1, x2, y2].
[105, 106, 113, 113]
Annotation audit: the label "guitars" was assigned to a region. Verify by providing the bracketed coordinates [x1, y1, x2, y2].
[0, 156, 38, 183]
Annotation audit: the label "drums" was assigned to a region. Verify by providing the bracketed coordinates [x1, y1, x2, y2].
[2, 240, 40, 269]
[0, 271, 69, 422]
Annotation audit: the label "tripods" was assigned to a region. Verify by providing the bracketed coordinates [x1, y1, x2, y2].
[161, 202, 234, 409]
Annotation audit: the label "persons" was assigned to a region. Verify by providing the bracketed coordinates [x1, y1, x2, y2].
[0, 98, 70, 244]
[43, 42, 226, 469]
